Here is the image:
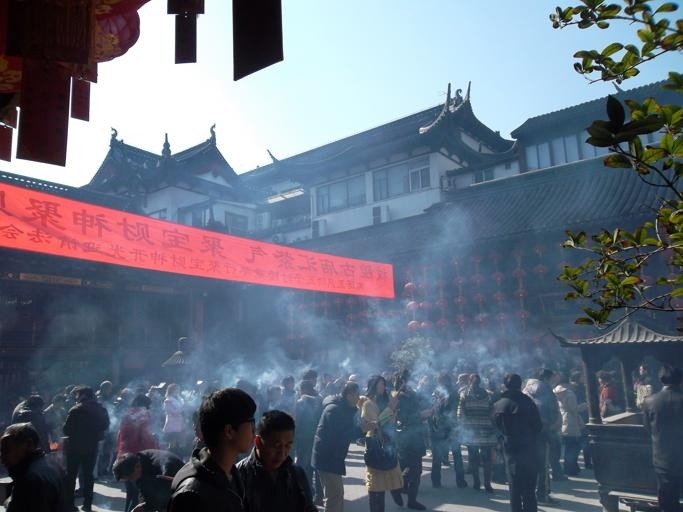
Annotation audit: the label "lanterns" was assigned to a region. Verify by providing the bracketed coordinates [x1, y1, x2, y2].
[287, 219, 681, 344]
[0, 0, 148, 166]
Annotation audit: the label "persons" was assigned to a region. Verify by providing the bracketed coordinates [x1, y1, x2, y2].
[1, 335, 683, 510]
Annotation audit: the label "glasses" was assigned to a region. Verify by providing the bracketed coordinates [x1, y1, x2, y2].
[241, 417, 255, 429]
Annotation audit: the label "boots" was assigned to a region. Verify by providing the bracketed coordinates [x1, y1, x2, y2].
[391, 487, 404, 506]
[407, 486, 425, 510]
[369, 491, 385, 512]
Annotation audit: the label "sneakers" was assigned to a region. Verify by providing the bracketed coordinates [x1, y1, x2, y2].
[433, 480, 441, 488]
[456, 480, 468, 488]
[536, 492, 561, 506]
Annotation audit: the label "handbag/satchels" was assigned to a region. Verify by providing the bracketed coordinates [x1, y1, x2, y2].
[362, 432, 398, 470]
